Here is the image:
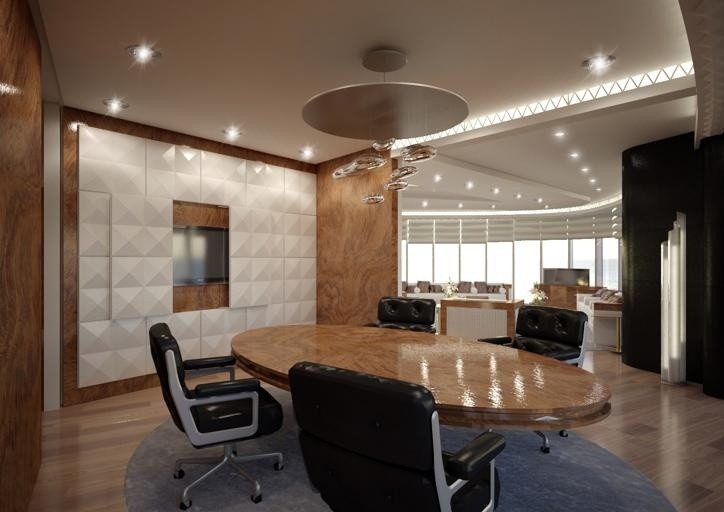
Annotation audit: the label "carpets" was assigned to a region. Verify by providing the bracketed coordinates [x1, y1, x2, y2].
[125, 386, 677, 512]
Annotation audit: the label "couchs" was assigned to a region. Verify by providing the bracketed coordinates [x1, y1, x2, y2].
[402, 280, 512, 301]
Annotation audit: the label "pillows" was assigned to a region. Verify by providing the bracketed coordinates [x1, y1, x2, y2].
[416, 281, 488, 293]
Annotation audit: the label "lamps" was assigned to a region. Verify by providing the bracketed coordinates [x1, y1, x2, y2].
[301, 42, 471, 207]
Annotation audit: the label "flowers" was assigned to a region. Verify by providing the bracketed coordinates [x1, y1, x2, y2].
[441, 279, 458, 297]
[527, 283, 549, 305]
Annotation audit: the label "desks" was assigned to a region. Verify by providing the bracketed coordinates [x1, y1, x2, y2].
[227, 321, 615, 435]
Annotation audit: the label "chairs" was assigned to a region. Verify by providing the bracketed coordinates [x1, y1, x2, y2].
[365, 296, 438, 334]
[288, 361, 509, 510]
[149, 322, 286, 511]
[475, 304, 590, 454]
